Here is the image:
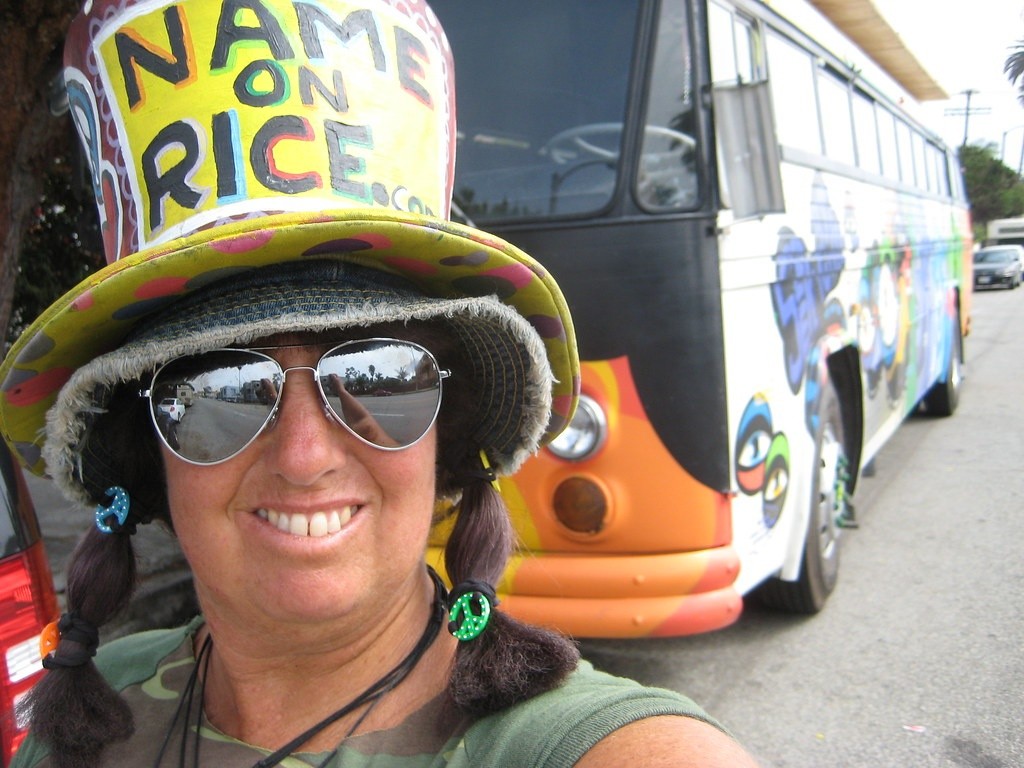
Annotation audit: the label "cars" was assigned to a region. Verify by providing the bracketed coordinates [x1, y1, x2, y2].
[974, 245, 1024, 290]
[372, 389, 392, 396]
[228, 393, 244, 404]
[153, 403, 181, 450]
[159, 398, 187, 421]
[0, 438, 63, 768]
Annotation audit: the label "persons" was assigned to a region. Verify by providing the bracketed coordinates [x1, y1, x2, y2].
[329, 373, 400, 449]
[260, 377, 278, 410]
[0, 272, 757, 768]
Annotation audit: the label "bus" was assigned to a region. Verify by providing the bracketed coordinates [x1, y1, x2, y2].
[427, 0, 973, 638]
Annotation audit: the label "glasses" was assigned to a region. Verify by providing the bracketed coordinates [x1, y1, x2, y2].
[140, 334, 453, 465]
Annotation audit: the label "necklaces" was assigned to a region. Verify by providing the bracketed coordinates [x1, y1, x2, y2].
[154, 560, 449, 768]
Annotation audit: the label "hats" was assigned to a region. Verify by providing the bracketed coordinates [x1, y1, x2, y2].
[43, 262, 554, 510]
[0, 0, 581, 480]
